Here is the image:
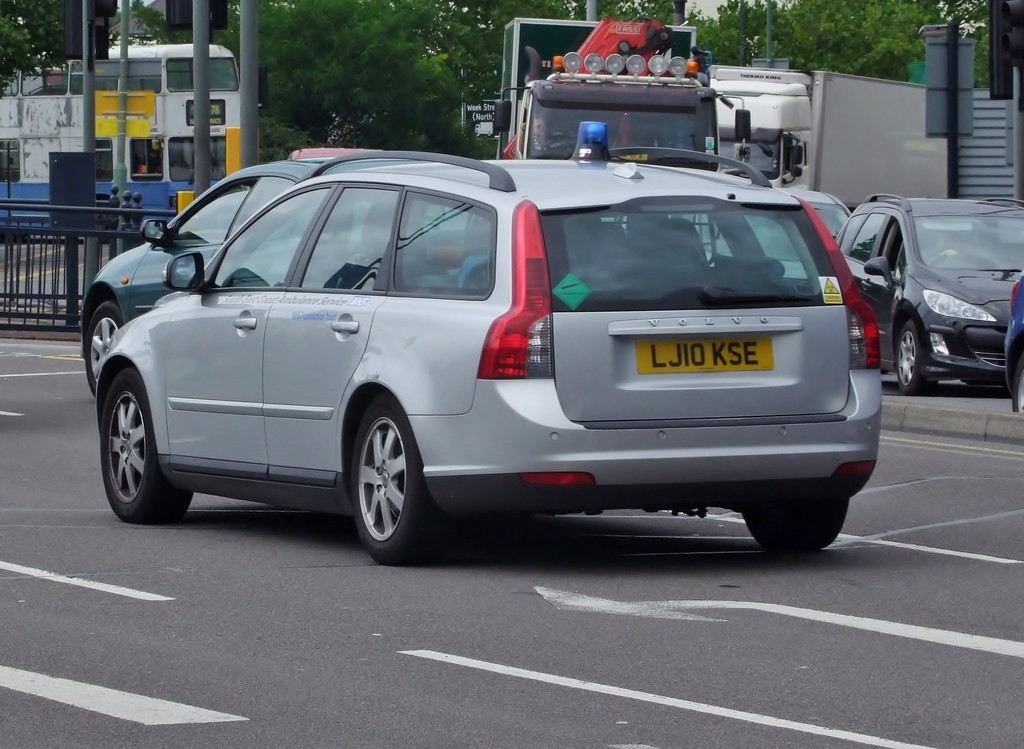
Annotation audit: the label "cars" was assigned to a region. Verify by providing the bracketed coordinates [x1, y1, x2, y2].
[82, 158, 446, 396]
[781, 187, 853, 245]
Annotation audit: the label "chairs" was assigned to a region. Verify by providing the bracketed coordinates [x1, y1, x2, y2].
[363, 202, 419, 272]
[643, 216, 708, 281]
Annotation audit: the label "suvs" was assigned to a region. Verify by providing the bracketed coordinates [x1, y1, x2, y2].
[834, 191, 1023, 396]
[93, 149, 882, 568]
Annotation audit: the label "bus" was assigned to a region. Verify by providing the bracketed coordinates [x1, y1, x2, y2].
[0, 43, 240, 245]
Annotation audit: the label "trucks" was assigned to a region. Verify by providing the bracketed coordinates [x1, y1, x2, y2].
[495, 16, 752, 164]
[710, 63, 946, 202]
